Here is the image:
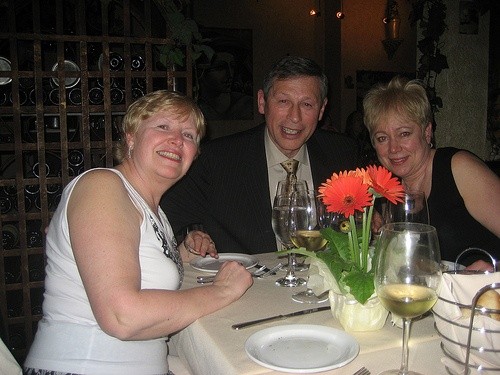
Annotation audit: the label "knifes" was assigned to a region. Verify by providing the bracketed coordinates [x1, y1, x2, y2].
[232, 305, 330, 330]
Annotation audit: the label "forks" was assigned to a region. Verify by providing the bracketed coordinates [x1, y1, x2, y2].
[196, 266, 265, 278]
[353, 367, 371, 375]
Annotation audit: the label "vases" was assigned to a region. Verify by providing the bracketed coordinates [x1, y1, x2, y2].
[328, 285, 395, 333]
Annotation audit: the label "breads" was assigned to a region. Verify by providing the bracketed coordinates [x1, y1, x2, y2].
[460, 289, 500, 322]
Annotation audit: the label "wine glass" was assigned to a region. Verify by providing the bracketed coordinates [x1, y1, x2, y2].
[271, 181, 312, 271]
[385, 190, 429, 229]
[317, 197, 344, 229]
[374, 222, 442, 374]
[289, 190, 329, 303]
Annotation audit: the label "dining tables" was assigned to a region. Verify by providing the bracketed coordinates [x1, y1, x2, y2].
[171, 251, 500, 375]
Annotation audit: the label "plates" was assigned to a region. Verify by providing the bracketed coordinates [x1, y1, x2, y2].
[191, 253, 258, 272]
[245, 325, 359, 372]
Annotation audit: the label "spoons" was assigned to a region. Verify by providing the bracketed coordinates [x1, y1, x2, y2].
[197, 263, 281, 283]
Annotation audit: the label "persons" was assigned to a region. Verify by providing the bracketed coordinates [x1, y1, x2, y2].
[363, 80, 500, 274]
[176, 57, 369, 254]
[22, 88, 254, 375]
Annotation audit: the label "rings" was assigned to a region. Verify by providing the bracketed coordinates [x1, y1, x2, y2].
[210, 241, 216, 248]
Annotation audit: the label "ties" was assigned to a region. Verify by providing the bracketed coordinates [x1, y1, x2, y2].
[280, 158, 300, 186]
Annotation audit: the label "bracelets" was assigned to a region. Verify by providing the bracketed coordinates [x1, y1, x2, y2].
[179, 223, 210, 235]
[184, 240, 201, 255]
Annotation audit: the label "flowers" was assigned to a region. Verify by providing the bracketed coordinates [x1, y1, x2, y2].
[275, 163, 406, 304]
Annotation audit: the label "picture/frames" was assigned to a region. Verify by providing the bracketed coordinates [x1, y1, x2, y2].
[458, 3, 479, 34]
